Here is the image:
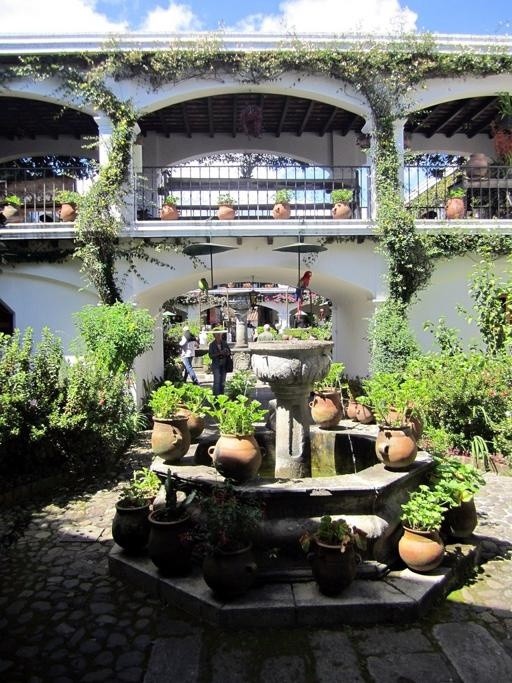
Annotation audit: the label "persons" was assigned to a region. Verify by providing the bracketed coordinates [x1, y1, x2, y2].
[258, 324, 274, 341]
[177, 326, 198, 385]
[208, 329, 231, 395]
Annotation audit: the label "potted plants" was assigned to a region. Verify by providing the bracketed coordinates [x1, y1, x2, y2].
[159, 195, 179, 220]
[272, 187, 292, 218]
[53, 189, 81, 221]
[217, 191, 237, 219]
[3, 194, 25, 222]
[445, 186, 493, 218]
[330, 188, 353, 219]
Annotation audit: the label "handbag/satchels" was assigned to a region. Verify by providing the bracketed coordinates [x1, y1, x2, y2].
[226, 355, 233, 372]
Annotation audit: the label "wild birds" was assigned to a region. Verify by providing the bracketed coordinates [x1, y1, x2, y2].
[198, 277, 208, 297]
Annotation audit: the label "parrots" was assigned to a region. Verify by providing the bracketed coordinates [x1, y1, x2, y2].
[294, 270, 314, 303]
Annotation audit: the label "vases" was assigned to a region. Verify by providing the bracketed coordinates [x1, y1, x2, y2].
[465, 151, 488, 177]
